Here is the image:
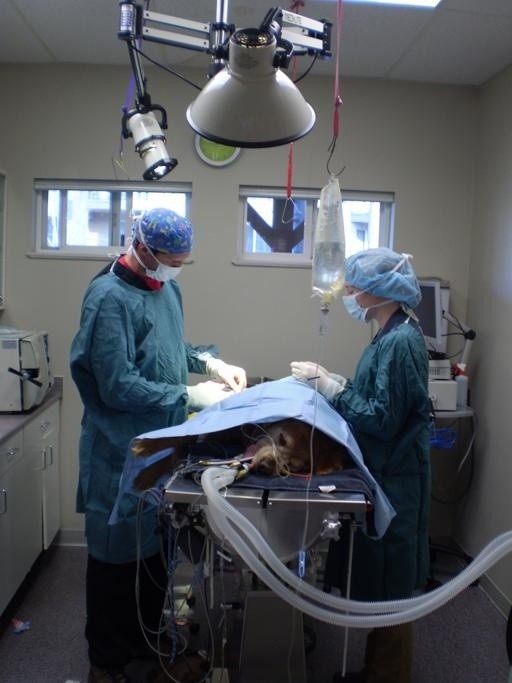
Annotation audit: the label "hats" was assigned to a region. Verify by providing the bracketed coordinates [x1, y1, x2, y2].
[132, 206, 193, 254]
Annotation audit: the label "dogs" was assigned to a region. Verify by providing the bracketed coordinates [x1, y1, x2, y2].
[131, 417, 340, 491]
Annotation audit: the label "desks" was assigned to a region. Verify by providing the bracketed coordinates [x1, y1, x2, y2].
[423, 407, 479, 594]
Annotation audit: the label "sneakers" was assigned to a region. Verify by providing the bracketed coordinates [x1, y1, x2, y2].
[88, 664, 133, 682]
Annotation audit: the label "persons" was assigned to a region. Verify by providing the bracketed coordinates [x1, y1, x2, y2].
[64, 204, 248, 678]
[283, 240, 436, 683]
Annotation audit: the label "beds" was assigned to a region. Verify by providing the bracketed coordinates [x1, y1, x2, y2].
[162, 456, 372, 654]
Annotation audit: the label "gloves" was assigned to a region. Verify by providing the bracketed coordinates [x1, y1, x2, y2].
[289, 359, 347, 402]
[182, 379, 234, 409]
[205, 353, 248, 393]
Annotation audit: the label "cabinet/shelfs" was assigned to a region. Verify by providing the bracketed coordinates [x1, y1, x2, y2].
[0, 399, 61, 615]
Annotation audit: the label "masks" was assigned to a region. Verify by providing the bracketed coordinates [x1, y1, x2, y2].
[137, 251, 184, 282]
[341, 285, 379, 323]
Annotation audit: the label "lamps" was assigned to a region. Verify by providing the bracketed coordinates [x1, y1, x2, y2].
[116, 0, 333, 183]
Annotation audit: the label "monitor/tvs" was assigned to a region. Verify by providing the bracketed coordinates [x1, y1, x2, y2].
[372, 279, 442, 354]
